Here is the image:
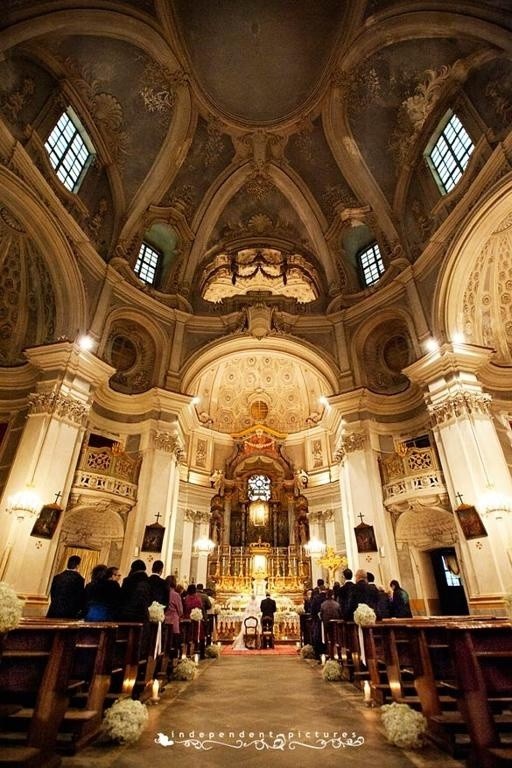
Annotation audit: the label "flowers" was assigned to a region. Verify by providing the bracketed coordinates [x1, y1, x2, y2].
[190, 607, 203, 624]
[208, 597, 221, 614]
[204, 643, 222, 658]
[301, 644, 316, 659]
[1, 580, 22, 631]
[148, 600, 166, 623]
[353, 603, 376, 627]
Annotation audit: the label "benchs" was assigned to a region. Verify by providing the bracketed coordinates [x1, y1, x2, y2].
[305, 615, 511, 768]
[1, 616, 215, 768]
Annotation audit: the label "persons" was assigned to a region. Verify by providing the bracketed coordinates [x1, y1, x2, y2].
[45, 555, 216, 678]
[232, 593, 277, 650]
[303, 569, 414, 659]
